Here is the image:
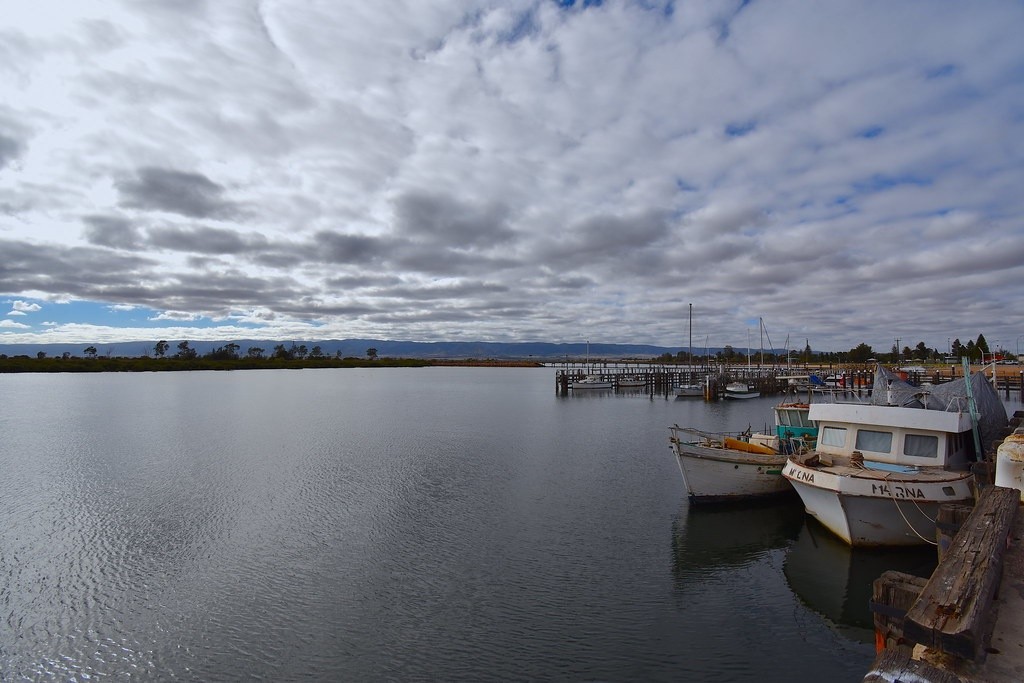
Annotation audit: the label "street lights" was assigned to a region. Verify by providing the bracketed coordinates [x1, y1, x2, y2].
[948, 337, 951, 357]
[806, 337, 809, 367]
[1017, 334, 1024, 360]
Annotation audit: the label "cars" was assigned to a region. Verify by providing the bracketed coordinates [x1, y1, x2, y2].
[981, 359, 1019, 365]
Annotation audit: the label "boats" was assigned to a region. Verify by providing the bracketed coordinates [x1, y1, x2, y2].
[775, 373, 829, 391]
[572, 341, 613, 389]
[674, 303, 721, 396]
[667, 379, 819, 505]
[771, 376, 872, 452]
[618, 378, 647, 386]
[723, 381, 760, 399]
[822, 375, 844, 388]
[780, 357, 1009, 547]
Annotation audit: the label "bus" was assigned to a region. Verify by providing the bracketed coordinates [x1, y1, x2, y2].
[945, 356, 958, 363]
